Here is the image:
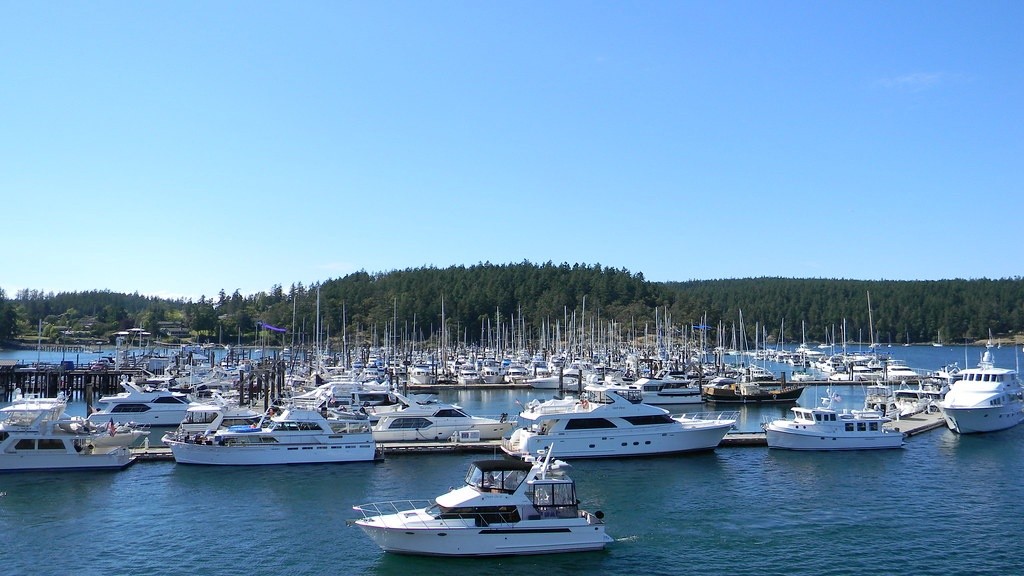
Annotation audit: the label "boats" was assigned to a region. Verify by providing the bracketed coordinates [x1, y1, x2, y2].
[0, 281, 929, 445]
[499, 381, 742, 458]
[349, 438, 616, 556]
[759, 381, 908, 448]
[0, 418, 140, 470]
[938, 327, 1024, 433]
[159, 403, 387, 467]
